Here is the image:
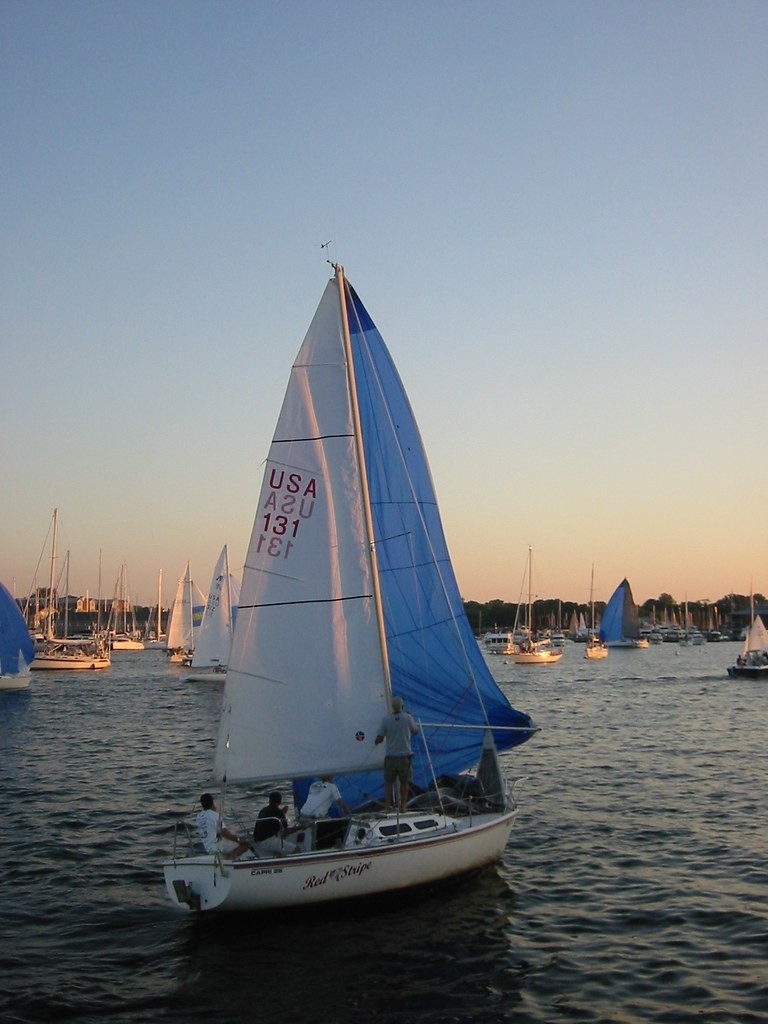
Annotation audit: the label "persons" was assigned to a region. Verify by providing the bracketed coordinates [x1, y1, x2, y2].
[375, 697, 418, 813]
[195, 792, 248, 860]
[299, 775, 357, 819]
[252, 791, 301, 855]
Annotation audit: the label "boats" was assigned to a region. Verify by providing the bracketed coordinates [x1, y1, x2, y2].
[631, 638, 649, 648]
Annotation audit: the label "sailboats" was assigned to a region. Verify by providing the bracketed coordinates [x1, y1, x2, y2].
[511, 545, 563, 663]
[585, 561, 608, 659]
[0, 581, 36, 691]
[22, 507, 110, 671]
[598, 577, 641, 647]
[22, 588, 45, 642]
[107, 563, 146, 650]
[166, 560, 207, 663]
[640, 605, 749, 645]
[678, 593, 693, 648]
[158, 260, 543, 921]
[139, 568, 166, 650]
[49, 550, 93, 646]
[726, 576, 768, 679]
[178, 544, 241, 681]
[474, 592, 599, 654]
